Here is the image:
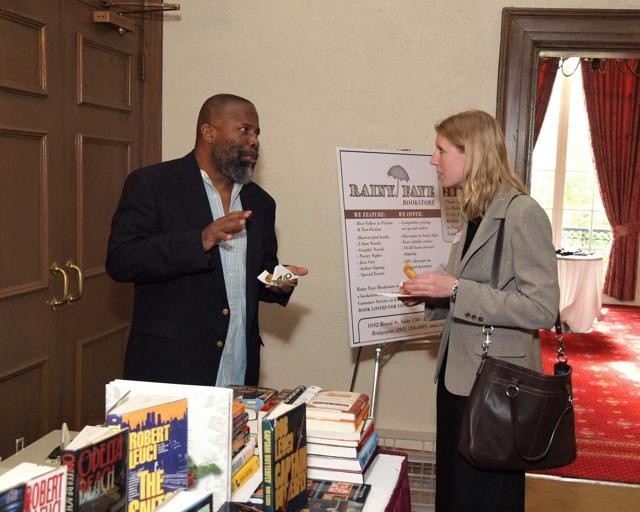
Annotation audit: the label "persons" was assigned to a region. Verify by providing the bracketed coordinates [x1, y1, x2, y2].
[394, 108, 564, 512]
[103, 95, 311, 395]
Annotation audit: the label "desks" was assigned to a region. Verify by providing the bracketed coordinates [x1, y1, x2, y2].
[524, 248, 606, 337]
[229, 447, 413, 512]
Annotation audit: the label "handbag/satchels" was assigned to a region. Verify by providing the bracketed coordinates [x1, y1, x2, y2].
[456, 355, 576, 474]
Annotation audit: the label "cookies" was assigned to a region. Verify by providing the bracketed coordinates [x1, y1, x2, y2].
[403, 263, 418, 280]
[265, 273, 273, 281]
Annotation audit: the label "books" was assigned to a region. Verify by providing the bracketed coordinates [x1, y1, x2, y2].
[1, 377, 382, 512]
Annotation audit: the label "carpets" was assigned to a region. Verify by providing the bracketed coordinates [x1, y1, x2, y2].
[524, 303, 640, 485]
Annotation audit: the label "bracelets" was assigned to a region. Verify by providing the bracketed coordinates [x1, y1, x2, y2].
[452, 280, 458, 299]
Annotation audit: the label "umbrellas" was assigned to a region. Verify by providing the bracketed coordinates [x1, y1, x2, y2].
[387, 164, 410, 197]
